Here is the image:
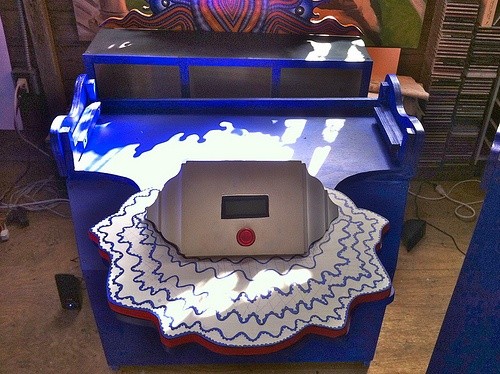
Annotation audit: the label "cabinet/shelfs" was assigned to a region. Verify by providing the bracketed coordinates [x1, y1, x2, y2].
[83, 28, 373, 101]
[51, 72, 427, 374]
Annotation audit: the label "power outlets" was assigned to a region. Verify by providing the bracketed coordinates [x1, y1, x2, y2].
[11, 67, 43, 96]
